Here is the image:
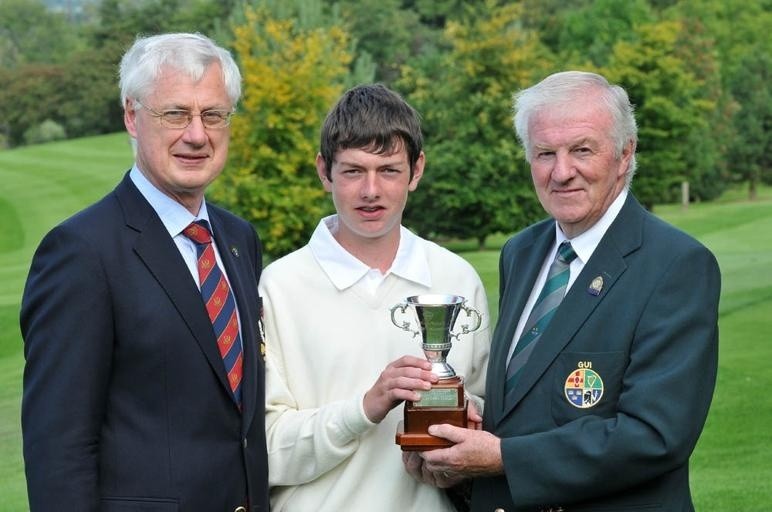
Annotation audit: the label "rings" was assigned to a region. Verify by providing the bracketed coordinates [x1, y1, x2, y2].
[440, 471, 450, 479]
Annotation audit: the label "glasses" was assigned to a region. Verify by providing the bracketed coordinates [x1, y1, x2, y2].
[135, 96, 234, 130]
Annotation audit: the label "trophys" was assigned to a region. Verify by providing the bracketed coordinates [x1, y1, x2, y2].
[382, 288, 485, 454]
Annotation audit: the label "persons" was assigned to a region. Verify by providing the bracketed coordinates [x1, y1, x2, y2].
[238, 79, 495, 511]
[16, 29, 273, 509]
[396, 67, 724, 511]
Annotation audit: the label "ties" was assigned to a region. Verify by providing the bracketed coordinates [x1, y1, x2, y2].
[180, 218, 244, 414]
[503, 239, 577, 415]
